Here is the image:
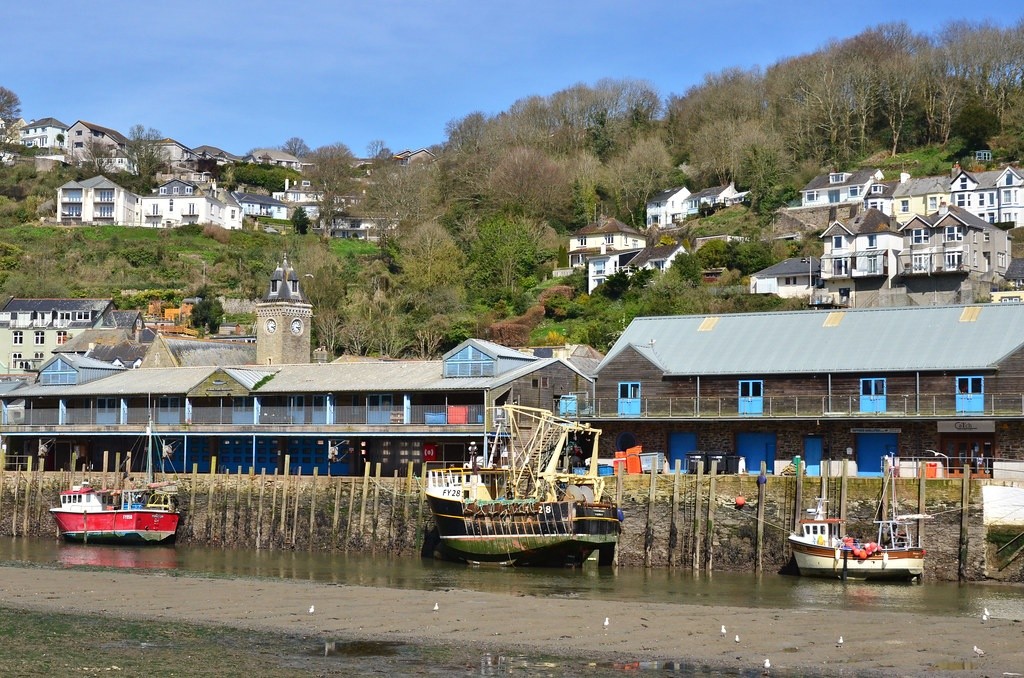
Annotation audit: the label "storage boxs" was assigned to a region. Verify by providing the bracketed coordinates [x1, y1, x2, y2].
[123, 503, 145, 510]
[571, 446, 663, 476]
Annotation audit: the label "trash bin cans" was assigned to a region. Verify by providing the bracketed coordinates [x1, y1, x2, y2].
[724, 456, 740, 474]
[686, 452, 707, 475]
[705, 451, 727, 475]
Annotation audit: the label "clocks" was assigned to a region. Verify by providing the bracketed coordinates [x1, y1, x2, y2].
[264, 318, 277, 335]
[290, 318, 304, 336]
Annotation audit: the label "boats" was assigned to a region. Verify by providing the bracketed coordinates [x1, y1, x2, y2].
[786, 466, 934, 581]
[47, 407, 186, 546]
[424, 400, 627, 567]
